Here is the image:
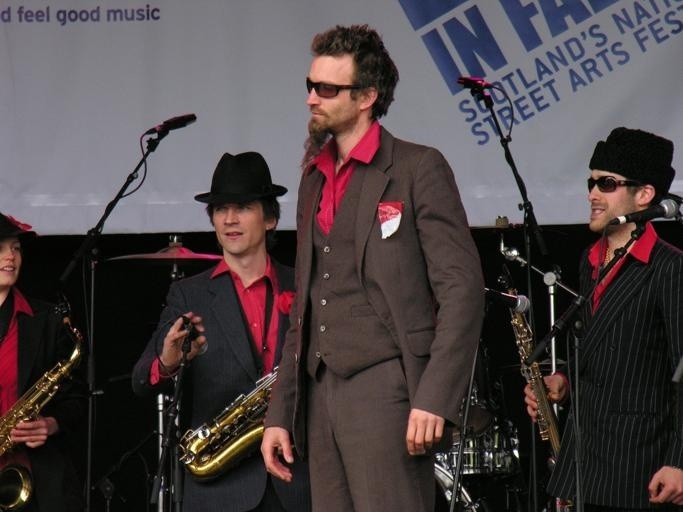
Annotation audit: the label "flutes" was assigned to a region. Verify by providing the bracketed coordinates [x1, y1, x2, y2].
[496, 260, 562, 460]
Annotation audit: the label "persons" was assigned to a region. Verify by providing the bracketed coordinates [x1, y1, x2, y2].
[131, 150, 311, 511]
[259, 23, 487, 511]
[523, 125, 683, 512]
[0, 213, 100, 511]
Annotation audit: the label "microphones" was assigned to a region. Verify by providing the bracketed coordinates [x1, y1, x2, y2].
[456, 77, 492, 95]
[181, 313, 207, 355]
[482, 285, 531, 314]
[608, 198, 678, 226]
[144, 112, 196, 136]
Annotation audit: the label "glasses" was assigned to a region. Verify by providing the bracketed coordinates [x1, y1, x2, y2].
[587, 175, 653, 193]
[305, 76, 372, 98]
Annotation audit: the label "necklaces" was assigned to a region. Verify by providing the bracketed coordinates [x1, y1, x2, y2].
[605, 248, 612, 271]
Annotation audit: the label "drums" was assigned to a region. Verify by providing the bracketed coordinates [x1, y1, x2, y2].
[445, 404, 519, 494]
[430, 462, 479, 512]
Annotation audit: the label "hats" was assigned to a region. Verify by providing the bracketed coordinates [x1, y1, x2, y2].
[0, 213, 37, 243]
[587, 125, 677, 195]
[193, 151, 289, 204]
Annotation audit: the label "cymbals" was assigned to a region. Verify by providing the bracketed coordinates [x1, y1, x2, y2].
[107, 246, 224, 268]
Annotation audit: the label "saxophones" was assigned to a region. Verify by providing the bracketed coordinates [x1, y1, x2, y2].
[180, 368, 274, 484]
[1, 285, 83, 512]
